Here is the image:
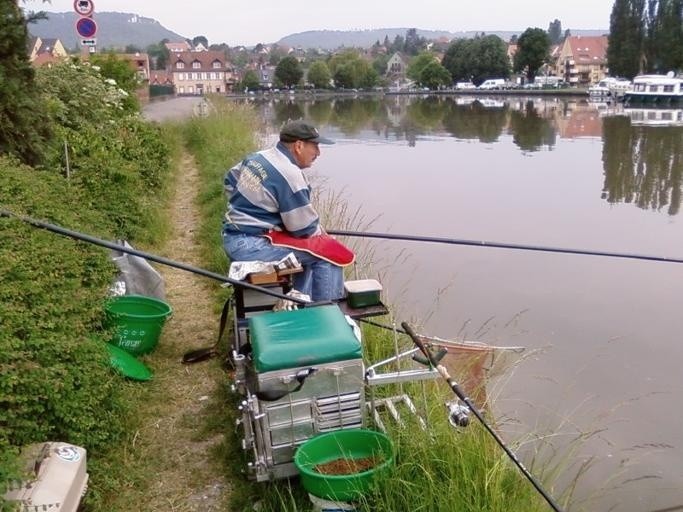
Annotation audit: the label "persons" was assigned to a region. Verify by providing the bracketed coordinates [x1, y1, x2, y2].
[221, 118, 345, 304]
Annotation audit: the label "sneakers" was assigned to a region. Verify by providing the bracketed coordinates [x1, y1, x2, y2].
[283, 289, 310, 304]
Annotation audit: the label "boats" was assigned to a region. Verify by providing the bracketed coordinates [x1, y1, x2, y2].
[589, 103, 683, 127]
[587, 71, 683, 102]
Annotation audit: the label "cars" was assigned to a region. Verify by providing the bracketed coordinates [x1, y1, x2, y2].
[506, 82, 552, 89]
[455, 97, 476, 105]
[456, 82, 476, 89]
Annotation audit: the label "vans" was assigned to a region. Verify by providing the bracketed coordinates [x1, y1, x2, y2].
[477, 98, 505, 108]
[478, 79, 504, 90]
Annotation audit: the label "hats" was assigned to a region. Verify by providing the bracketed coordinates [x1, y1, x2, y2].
[279, 120, 335, 145]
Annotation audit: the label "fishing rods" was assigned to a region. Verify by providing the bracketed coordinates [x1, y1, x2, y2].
[2, 210, 525, 350]
[401, 320, 563, 512]
[325, 230, 683, 264]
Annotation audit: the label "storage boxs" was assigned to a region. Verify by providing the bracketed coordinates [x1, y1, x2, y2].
[5, 441, 90, 512]
[344, 280, 384, 309]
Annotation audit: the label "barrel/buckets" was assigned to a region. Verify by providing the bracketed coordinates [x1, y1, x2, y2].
[103, 294, 172, 354]
